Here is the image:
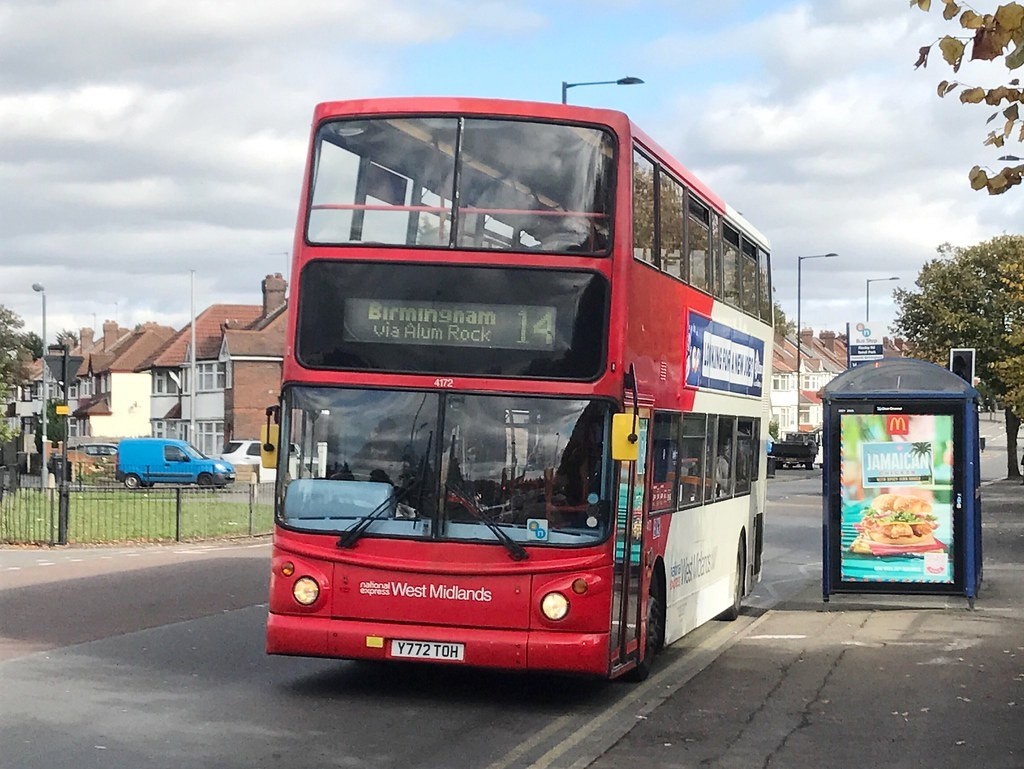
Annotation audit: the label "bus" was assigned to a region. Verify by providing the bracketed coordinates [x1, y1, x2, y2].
[261, 96, 777, 684]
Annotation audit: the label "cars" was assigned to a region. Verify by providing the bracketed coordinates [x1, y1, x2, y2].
[68, 443, 118, 457]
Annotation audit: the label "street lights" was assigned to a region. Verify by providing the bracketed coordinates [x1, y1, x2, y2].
[797, 253, 839, 434]
[866, 277, 900, 324]
[32, 283, 48, 491]
[562, 76, 645, 106]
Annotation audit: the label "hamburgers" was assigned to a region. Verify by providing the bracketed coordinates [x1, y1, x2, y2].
[852, 492, 935, 545]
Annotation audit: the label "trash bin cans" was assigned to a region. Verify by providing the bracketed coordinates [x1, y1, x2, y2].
[51, 456, 63, 483]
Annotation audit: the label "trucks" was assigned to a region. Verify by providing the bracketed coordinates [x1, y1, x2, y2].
[770, 431, 821, 471]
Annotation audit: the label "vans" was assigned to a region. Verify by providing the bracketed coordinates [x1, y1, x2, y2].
[117, 438, 237, 490]
[219, 439, 277, 482]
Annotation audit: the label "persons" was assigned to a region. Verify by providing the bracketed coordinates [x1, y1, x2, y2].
[349, 418, 404, 470]
[716, 438, 732, 497]
